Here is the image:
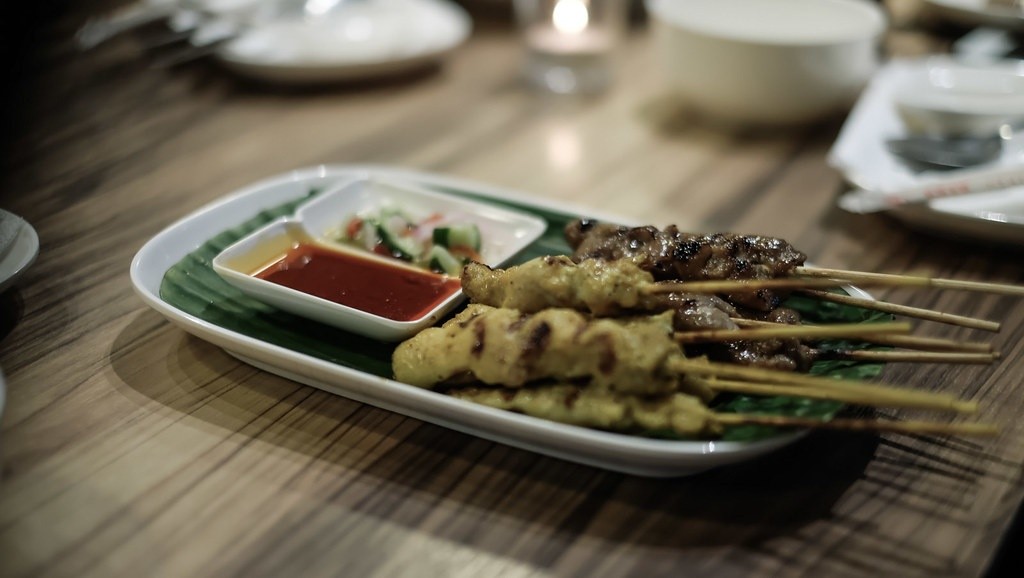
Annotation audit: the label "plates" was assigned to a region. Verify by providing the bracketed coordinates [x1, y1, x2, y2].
[828, 52, 1023, 249]
[214, 175, 551, 345]
[200, 0, 479, 82]
[126, 161, 895, 476]
[1, 206, 39, 297]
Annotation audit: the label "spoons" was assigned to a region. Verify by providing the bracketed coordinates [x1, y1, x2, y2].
[884, 116, 1022, 172]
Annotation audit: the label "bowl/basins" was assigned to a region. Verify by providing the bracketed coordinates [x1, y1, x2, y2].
[644, 0, 887, 140]
